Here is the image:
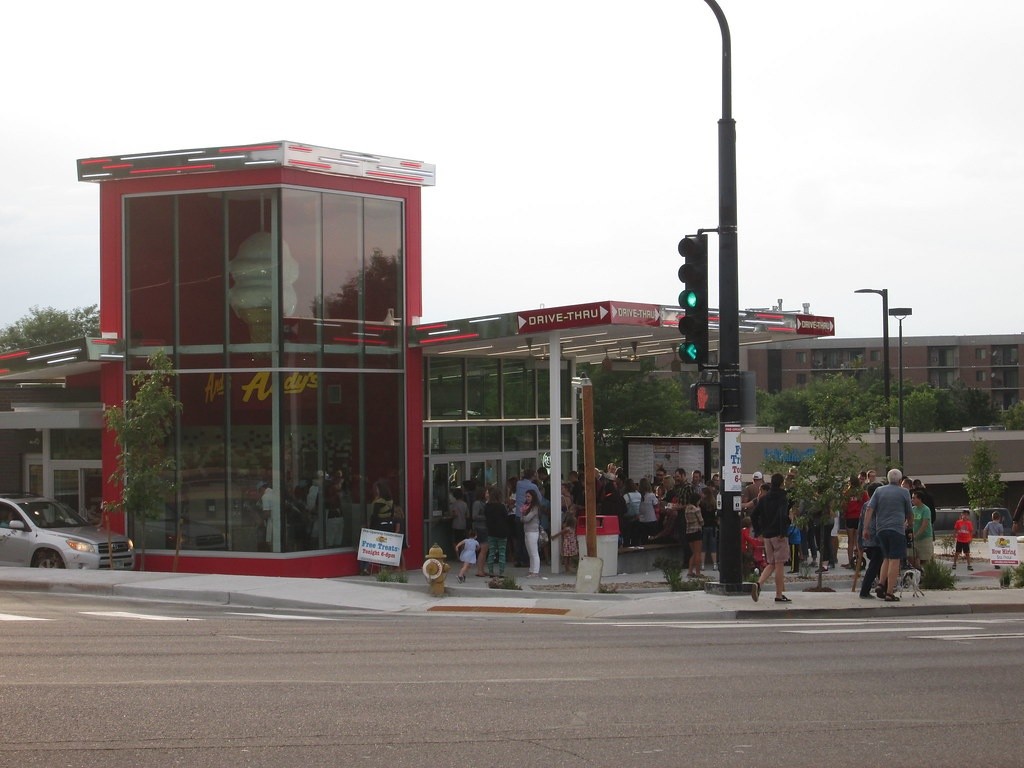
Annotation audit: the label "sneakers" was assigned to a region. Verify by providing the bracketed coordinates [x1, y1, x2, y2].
[774, 595, 792, 603]
[815, 566, 830, 573]
[751, 582, 761, 602]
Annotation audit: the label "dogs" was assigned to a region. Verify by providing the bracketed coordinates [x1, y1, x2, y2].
[899, 565, 926, 598]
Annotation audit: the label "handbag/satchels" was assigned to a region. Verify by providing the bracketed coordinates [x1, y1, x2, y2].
[622, 502, 637, 521]
[538, 524, 549, 545]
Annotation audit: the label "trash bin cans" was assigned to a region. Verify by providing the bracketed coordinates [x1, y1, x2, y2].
[577, 515, 621, 577]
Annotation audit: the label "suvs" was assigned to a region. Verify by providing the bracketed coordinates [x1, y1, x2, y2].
[0, 491, 135, 571]
[128, 493, 226, 550]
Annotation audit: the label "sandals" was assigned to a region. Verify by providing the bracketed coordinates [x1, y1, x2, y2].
[884, 593, 900, 601]
[874, 584, 887, 599]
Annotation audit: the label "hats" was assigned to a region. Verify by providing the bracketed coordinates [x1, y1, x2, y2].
[752, 472, 763, 480]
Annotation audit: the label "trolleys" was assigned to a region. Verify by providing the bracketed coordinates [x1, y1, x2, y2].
[744, 546, 767, 576]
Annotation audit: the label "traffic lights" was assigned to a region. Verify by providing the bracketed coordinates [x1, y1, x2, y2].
[688, 383, 724, 413]
[678, 234, 709, 365]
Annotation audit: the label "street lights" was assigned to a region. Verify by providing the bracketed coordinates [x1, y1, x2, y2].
[888, 307, 913, 478]
[853, 288, 893, 478]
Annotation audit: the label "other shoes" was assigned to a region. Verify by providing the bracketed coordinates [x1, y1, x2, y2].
[841, 563, 856, 570]
[952, 564, 957, 570]
[456, 575, 466, 584]
[523, 573, 540, 578]
[474, 571, 490, 578]
[966, 566, 974, 570]
[700, 565, 706, 571]
[490, 573, 496, 579]
[787, 570, 799, 576]
[859, 593, 877, 599]
[685, 573, 707, 579]
[499, 575, 508, 580]
[712, 564, 718, 571]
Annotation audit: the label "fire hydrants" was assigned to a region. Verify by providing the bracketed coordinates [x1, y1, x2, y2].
[422, 544, 452, 595]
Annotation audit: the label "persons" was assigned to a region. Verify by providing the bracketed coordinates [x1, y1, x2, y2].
[456, 528, 481, 584]
[861, 468, 915, 601]
[752, 473, 794, 604]
[431, 460, 937, 604]
[684, 492, 705, 578]
[952, 509, 974, 571]
[519, 489, 543, 579]
[983, 510, 1004, 569]
[362, 478, 410, 576]
[245, 467, 352, 553]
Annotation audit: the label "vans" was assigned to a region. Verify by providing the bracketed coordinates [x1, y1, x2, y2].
[932, 505, 1013, 540]
